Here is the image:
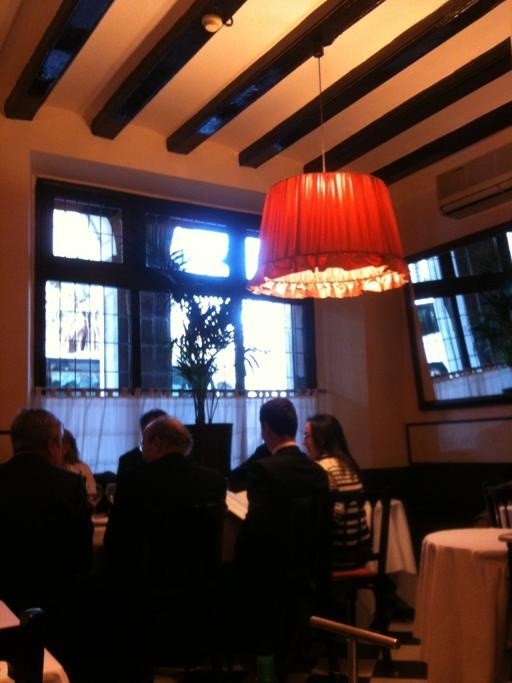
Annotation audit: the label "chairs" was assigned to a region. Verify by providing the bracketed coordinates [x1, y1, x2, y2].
[482, 479, 512, 526]
[0, 608, 48, 683]
[331, 488, 396, 673]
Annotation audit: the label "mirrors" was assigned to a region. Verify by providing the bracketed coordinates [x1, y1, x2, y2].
[402, 220, 512, 411]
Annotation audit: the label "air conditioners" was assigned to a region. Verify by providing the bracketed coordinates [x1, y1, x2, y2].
[436, 138, 512, 219]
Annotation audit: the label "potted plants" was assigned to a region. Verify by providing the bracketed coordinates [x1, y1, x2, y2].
[131, 224, 233, 468]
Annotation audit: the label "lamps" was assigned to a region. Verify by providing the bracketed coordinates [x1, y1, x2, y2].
[246, 48, 407, 300]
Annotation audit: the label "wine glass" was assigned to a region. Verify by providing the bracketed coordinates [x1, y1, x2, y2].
[85, 483, 116, 519]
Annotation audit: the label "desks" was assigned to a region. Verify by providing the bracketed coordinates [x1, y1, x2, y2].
[0, 596, 71, 683]
[92, 493, 412, 577]
[414, 528, 512, 682]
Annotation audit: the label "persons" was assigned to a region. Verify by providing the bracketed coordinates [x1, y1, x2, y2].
[61, 427, 99, 512]
[229, 440, 273, 493]
[224, 396, 336, 681]
[100, 414, 229, 683]
[112, 407, 166, 505]
[301, 412, 372, 652]
[1, 407, 100, 683]
